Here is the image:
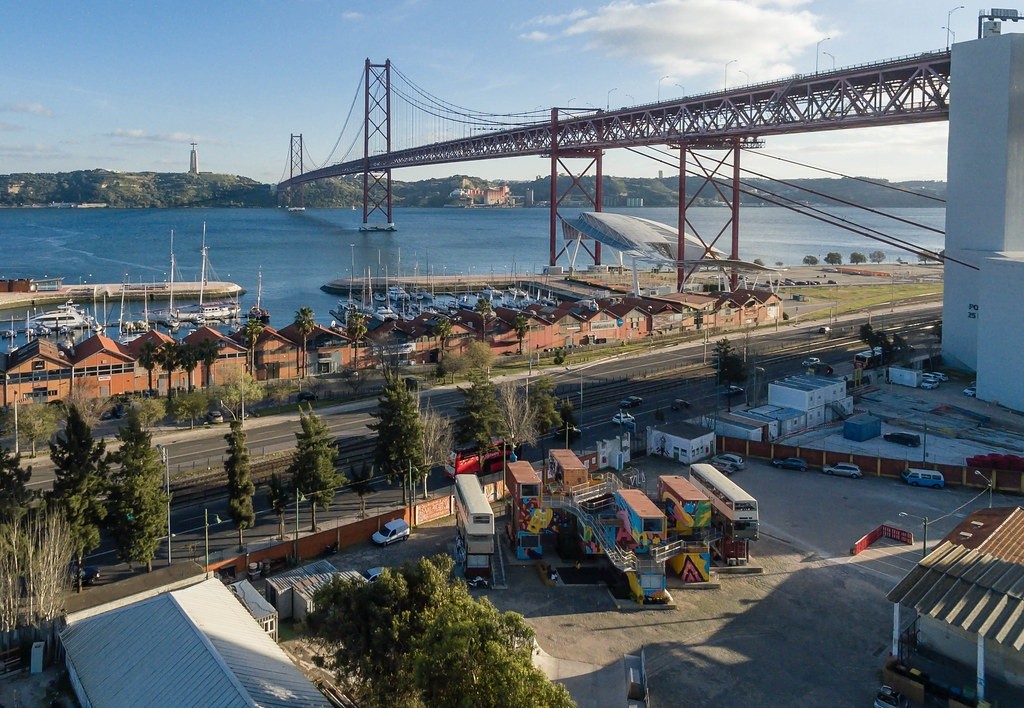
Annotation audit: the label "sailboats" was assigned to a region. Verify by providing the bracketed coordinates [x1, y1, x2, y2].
[142, 220, 242, 321]
[357, 248, 560, 321]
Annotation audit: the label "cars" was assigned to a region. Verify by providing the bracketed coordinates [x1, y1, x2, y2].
[723, 385, 744, 397]
[809, 362, 833, 376]
[554, 424, 581, 442]
[620, 395, 642, 408]
[822, 461, 863, 479]
[802, 357, 820, 368]
[883, 432, 921, 447]
[770, 456, 809, 472]
[612, 413, 635, 425]
[964, 386, 976, 397]
[921, 372, 949, 390]
[671, 398, 691, 410]
[898, 345, 915, 352]
[364, 566, 392, 582]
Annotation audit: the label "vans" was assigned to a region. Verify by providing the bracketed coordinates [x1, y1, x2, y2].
[901, 468, 944, 489]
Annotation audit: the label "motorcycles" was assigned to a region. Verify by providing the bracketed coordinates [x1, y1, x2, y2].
[467, 576, 489, 589]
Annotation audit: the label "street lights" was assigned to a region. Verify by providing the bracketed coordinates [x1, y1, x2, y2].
[975, 470, 992, 508]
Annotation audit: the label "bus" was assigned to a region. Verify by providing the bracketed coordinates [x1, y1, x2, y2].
[853, 347, 882, 370]
[444, 436, 523, 481]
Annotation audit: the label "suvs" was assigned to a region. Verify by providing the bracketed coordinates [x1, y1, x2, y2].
[819, 327, 832, 334]
[371, 518, 411, 547]
[701, 454, 745, 476]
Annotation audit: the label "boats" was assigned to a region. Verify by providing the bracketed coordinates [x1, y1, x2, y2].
[25, 270, 148, 345]
